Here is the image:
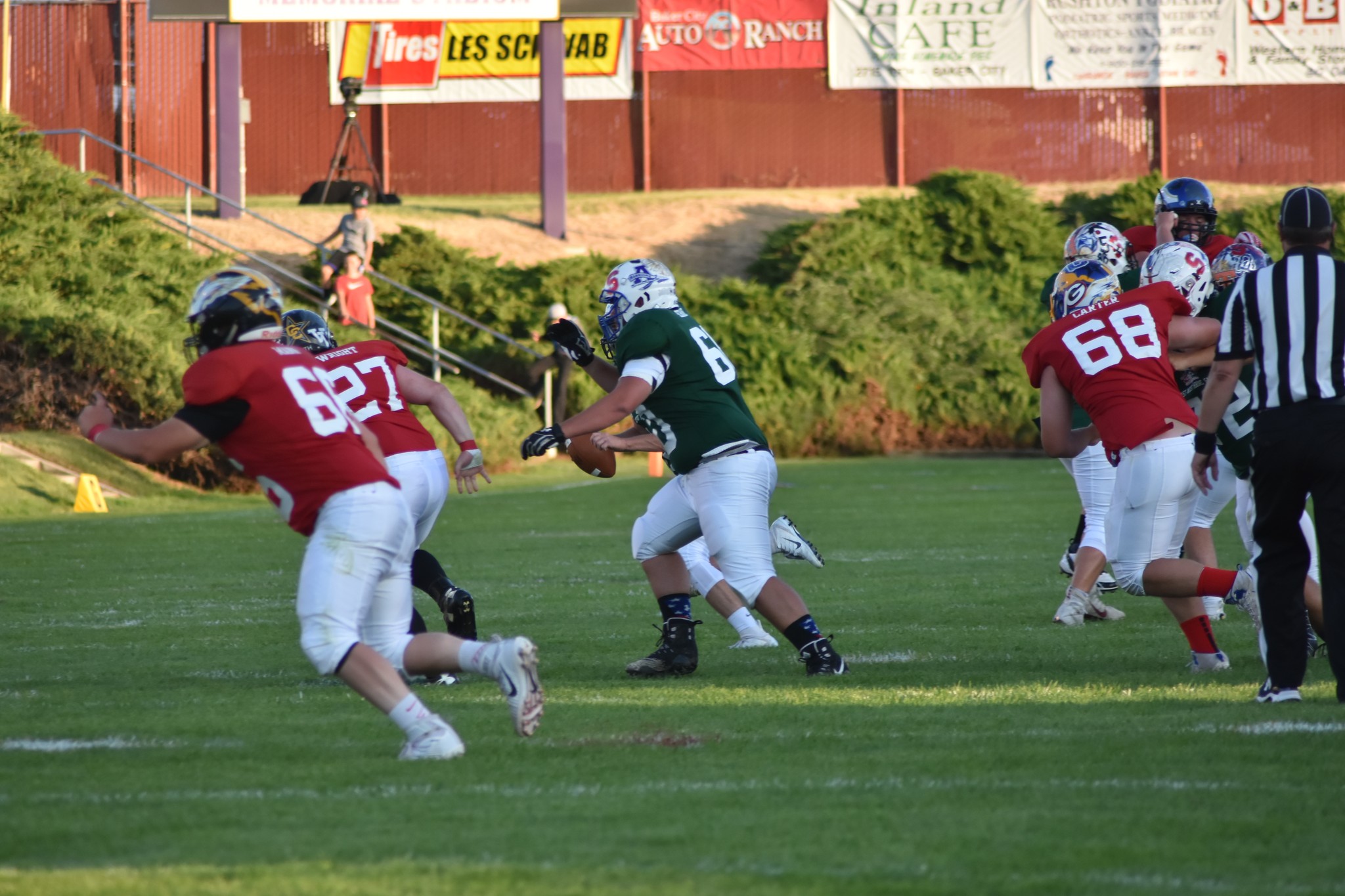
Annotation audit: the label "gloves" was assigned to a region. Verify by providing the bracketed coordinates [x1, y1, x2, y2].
[539, 318, 597, 366]
[520, 422, 566, 461]
[1234, 231, 1263, 249]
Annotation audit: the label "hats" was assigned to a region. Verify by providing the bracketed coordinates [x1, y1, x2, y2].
[352, 196, 371, 209]
[1279, 186, 1332, 228]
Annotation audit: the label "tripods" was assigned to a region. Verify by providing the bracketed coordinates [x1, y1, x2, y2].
[320, 100, 385, 206]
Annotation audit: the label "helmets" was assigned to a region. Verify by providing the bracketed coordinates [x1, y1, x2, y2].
[1137, 241, 1214, 318]
[1211, 243, 1273, 291]
[1050, 258, 1122, 323]
[273, 309, 337, 355]
[1064, 222, 1134, 276]
[183, 267, 285, 345]
[1153, 177, 1217, 229]
[604, 259, 679, 327]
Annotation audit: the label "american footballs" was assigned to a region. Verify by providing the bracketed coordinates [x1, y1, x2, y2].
[564, 431, 616, 479]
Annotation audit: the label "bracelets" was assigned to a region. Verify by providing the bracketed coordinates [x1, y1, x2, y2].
[458, 440, 478, 452]
[88, 423, 109, 442]
[1194, 428, 1216, 455]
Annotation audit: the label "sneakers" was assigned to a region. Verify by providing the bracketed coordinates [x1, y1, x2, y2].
[728, 632, 779, 649]
[1058, 549, 1119, 594]
[1254, 674, 1302, 705]
[1052, 599, 1086, 627]
[1222, 565, 1264, 638]
[439, 586, 478, 642]
[1184, 649, 1230, 674]
[395, 724, 465, 764]
[770, 515, 825, 570]
[400, 668, 462, 686]
[1208, 614, 1227, 621]
[1083, 596, 1127, 623]
[495, 637, 547, 738]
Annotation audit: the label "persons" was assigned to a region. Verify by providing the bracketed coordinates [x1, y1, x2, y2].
[530, 301, 589, 426]
[1020, 177, 1328, 674]
[336, 252, 376, 337]
[522, 255, 849, 679]
[275, 308, 493, 689]
[77, 268, 544, 760]
[1191, 184, 1345, 707]
[315, 196, 374, 296]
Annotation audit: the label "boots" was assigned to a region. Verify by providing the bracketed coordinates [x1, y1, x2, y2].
[624, 617, 704, 677]
[797, 633, 851, 676]
